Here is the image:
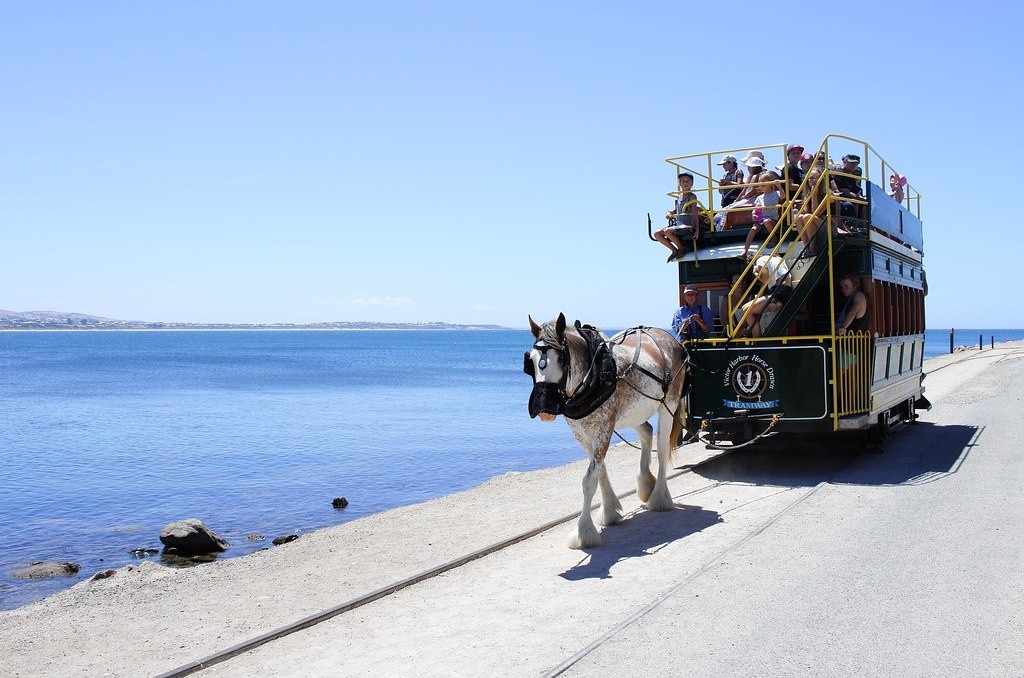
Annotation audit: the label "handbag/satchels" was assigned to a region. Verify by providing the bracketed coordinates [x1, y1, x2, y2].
[721, 188, 741, 206]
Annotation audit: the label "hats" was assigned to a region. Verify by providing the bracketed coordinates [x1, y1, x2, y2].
[741, 150, 764, 162]
[786, 144, 804, 156]
[842, 154, 860, 164]
[800, 153, 814, 163]
[855, 166, 862, 173]
[717, 154, 737, 165]
[889, 173, 907, 190]
[742, 157, 763, 167]
[677, 172, 693, 180]
[683, 284, 699, 294]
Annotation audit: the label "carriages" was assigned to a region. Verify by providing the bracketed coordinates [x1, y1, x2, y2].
[520, 131, 934, 547]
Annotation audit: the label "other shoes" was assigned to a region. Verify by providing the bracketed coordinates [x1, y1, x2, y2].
[799, 249, 819, 257]
[766, 238, 779, 249]
[676, 246, 686, 259]
[737, 251, 748, 260]
[667, 251, 679, 263]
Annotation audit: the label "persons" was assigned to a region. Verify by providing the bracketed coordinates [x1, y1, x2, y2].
[671, 285, 714, 340]
[654, 173, 699, 263]
[717, 145, 906, 344]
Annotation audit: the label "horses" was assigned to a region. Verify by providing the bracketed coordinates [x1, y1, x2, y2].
[528, 312, 686, 549]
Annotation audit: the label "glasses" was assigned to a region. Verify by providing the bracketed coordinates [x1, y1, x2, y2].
[817, 156, 825, 161]
[731, 279, 746, 285]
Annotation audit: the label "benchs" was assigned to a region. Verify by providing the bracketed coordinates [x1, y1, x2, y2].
[668, 217, 780, 237]
